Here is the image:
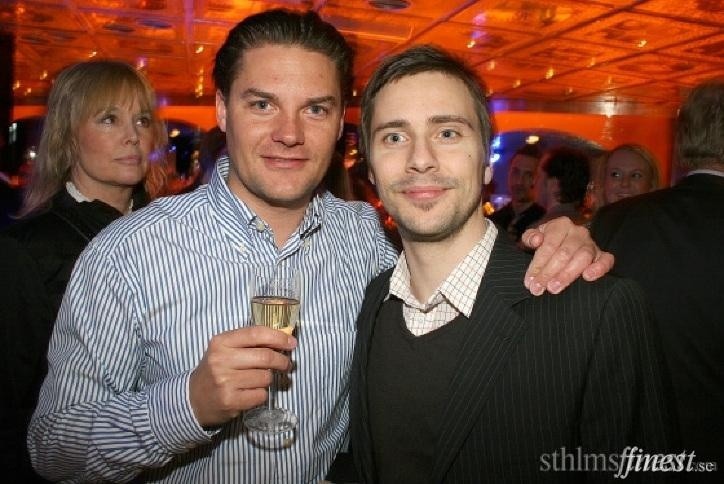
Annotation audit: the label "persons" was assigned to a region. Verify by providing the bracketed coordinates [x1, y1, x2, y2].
[26, 6, 621, 484]
[347, 42, 687, 482]
[589, 77, 723, 483]
[1, 59, 163, 482]
[174, 125, 228, 197]
[484, 141, 663, 239]
[314, 115, 404, 255]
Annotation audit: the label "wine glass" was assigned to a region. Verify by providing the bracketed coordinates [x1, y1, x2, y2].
[240, 263, 303, 435]
